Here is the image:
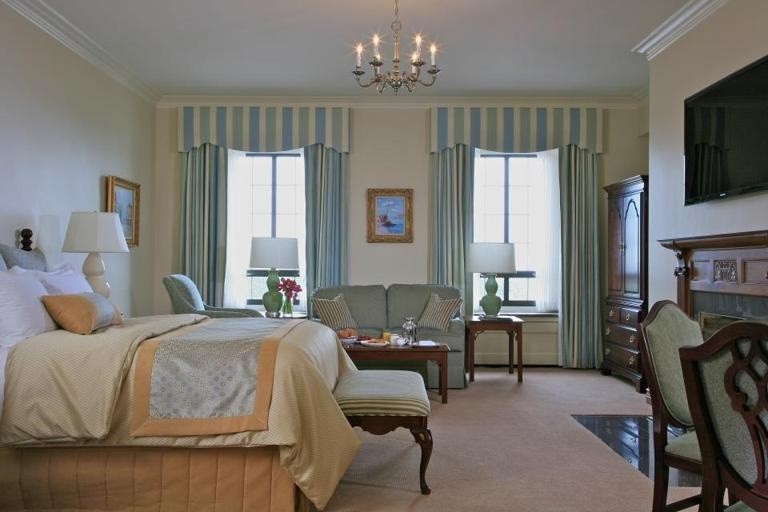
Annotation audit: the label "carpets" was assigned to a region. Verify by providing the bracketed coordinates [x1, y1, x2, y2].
[310, 367, 730, 512]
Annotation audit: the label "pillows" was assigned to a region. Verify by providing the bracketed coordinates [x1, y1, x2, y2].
[417, 292, 463, 333]
[0, 246, 124, 346]
[311, 292, 358, 331]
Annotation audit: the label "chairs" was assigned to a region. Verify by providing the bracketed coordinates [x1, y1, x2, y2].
[163, 273, 264, 318]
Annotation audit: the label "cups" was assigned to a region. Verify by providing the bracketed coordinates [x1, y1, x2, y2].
[383, 329, 408, 348]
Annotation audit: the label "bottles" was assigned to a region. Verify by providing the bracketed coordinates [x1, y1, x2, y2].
[402, 317, 419, 347]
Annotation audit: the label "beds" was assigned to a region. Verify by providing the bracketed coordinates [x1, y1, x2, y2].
[0, 227, 337, 512]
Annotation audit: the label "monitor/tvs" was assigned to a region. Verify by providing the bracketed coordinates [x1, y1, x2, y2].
[682, 54, 768, 206]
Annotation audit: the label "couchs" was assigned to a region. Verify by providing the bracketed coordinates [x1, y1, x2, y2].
[309, 284, 467, 390]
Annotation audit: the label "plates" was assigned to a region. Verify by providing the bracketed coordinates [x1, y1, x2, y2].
[356, 335, 387, 348]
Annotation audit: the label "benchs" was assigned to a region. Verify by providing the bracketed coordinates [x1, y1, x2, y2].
[332, 369, 433, 495]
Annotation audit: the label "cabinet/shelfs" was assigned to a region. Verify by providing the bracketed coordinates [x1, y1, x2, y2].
[636, 297, 740, 512]
[600, 173, 648, 394]
[656, 229, 768, 433]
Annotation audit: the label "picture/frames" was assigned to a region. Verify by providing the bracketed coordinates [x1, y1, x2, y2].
[367, 187, 414, 244]
[106, 174, 142, 248]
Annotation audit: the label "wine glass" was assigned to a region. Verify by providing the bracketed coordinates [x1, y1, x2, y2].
[338, 335, 358, 350]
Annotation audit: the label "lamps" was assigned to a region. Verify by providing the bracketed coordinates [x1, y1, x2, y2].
[61, 210, 131, 299]
[246, 237, 300, 313]
[465, 243, 517, 318]
[352, 0, 442, 97]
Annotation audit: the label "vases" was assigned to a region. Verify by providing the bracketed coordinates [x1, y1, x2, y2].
[283, 298, 293, 313]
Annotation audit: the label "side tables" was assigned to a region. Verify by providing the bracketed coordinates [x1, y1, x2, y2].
[465, 315, 524, 383]
[260, 310, 309, 319]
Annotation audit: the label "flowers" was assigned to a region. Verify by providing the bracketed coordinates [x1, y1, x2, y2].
[276, 277, 302, 300]
